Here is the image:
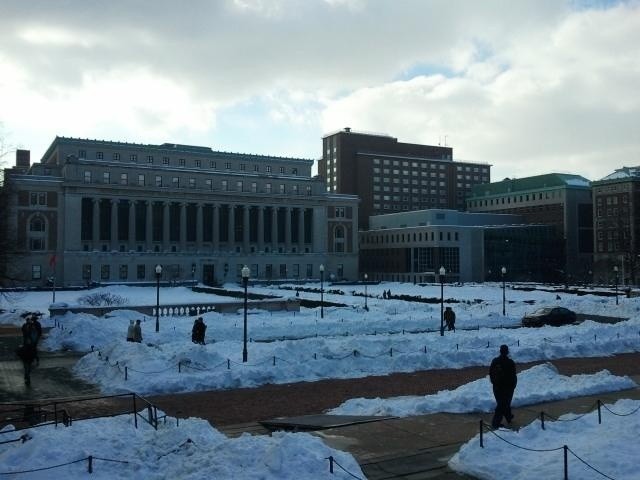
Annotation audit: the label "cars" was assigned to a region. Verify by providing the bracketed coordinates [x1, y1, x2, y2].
[522, 306, 578, 327]
[544, 270, 573, 284]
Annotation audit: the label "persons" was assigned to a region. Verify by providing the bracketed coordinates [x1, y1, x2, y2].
[625, 285, 632, 298]
[22, 316, 41, 365]
[444, 306, 457, 331]
[490, 344, 517, 429]
[387, 289, 392, 299]
[192, 317, 207, 345]
[556, 294, 560, 301]
[383, 290, 386, 299]
[127, 319, 143, 343]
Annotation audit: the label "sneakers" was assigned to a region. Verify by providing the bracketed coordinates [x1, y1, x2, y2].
[508, 415, 513, 423]
[493, 424, 504, 428]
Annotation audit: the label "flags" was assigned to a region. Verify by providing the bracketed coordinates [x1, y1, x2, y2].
[48, 255, 57, 273]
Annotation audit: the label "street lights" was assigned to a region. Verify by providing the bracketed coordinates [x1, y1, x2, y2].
[613, 265, 619, 307]
[242, 264, 250, 362]
[363, 275, 370, 312]
[439, 267, 445, 338]
[501, 267, 506, 319]
[156, 266, 162, 333]
[319, 263, 327, 318]
